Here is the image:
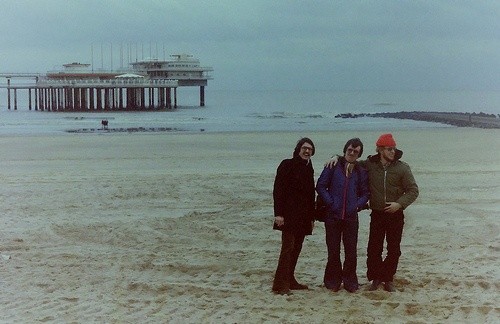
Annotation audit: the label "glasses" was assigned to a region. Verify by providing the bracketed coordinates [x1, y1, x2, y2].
[348, 148, 360, 154]
[300, 147, 313, 153]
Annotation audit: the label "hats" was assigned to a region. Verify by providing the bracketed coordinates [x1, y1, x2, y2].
[376, 133, 396, 147]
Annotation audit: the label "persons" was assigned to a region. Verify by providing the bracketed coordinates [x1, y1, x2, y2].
[271, 137, 315, 293]
[315, 137, 369, 293]
[325, 133, 419, 291]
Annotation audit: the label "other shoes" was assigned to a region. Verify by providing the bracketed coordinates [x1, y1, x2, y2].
[347, 286, 357, 293]
[332, 286, 340, 292]
[274, 289, 293, 295]
[385, 280, 396, 292]
[372, 280, 382, 289]
[290, 282, 309, 289]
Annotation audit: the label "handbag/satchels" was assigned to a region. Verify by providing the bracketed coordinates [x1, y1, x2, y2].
[314, 198, 329, 222]
